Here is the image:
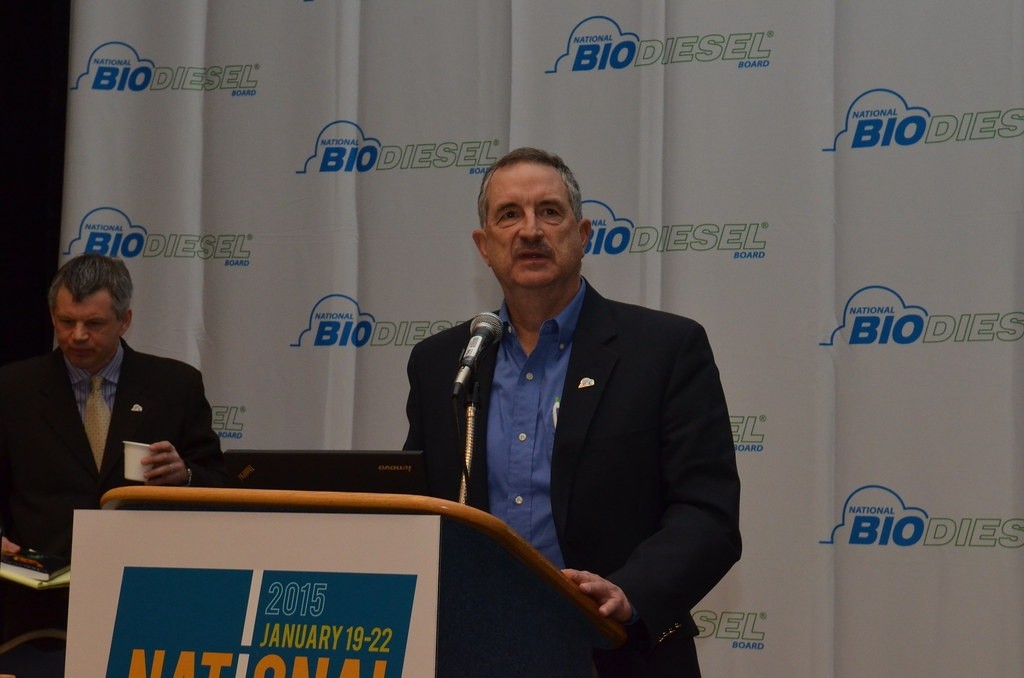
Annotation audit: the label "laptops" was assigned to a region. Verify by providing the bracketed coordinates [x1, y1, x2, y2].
[222, 448, 424, 494]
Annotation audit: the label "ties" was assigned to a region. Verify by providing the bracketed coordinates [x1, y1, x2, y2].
[82, 375, 111, 473]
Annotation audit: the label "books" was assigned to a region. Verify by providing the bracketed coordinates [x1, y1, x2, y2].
[0, 545, 71, 581]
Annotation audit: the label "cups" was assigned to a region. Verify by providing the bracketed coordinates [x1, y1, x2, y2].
[122, 441, 158, 481]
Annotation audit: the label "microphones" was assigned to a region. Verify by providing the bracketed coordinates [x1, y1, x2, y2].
[450, 311, 504, 400]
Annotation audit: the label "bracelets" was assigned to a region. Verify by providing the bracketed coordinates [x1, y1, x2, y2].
[186, 468, 192, 487]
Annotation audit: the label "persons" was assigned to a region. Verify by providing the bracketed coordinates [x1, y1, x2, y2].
[401, 147, 742, 677]
[1, 252, 236, 677]
